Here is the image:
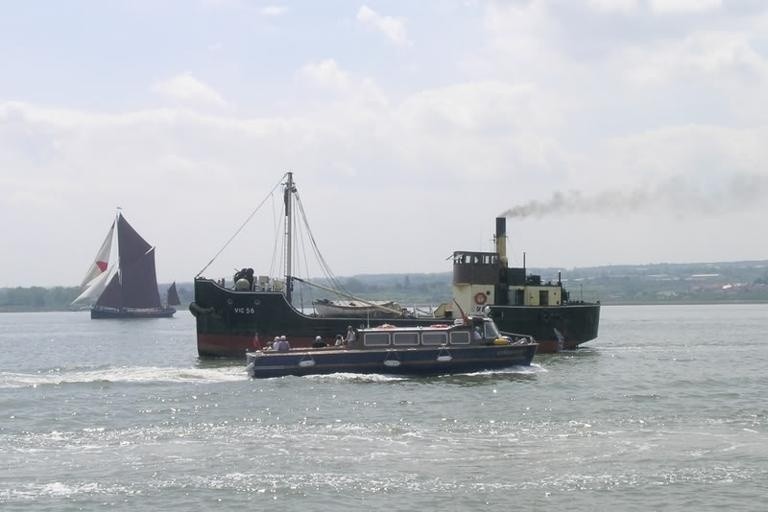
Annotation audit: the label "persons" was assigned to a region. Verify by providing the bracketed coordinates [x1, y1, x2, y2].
[334, 336, 343, 345]
[312, 335, 329, 347]
[263, 335, 289, 351]
[473, 326, 481, 340]
[345, 325, 357, 343]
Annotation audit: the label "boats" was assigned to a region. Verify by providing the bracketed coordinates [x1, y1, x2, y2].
[244, 316, 539, 379]
[188, 171, 600, 359]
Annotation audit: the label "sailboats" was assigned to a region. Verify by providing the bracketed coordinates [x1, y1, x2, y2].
[69, 206, 180, 319]
